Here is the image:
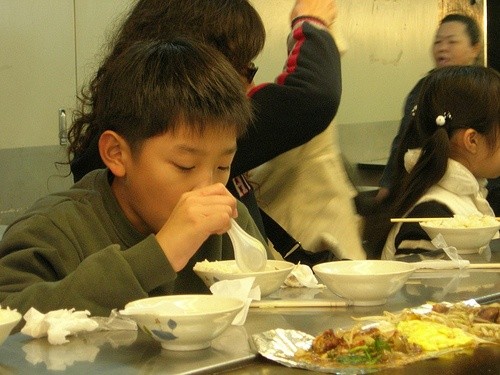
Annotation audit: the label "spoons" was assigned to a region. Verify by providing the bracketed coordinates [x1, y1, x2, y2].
[225, 217, 268, 271]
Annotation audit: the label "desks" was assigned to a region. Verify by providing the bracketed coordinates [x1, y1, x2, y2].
[0, 239, 500, 375]
[358, 159, 388, 172]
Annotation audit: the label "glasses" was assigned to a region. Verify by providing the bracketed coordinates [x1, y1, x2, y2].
[234, 61, 259, 84]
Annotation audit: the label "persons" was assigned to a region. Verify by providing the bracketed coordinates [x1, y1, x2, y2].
[0, 38, 277, 317]
[368, 14, 481, 204]
[366, 66, 500, 263]
[46, 0, 353, 268]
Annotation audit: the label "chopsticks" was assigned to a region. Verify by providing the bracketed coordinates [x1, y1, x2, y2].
[391, 217, 499, 222]
[463, 263, 500, 269]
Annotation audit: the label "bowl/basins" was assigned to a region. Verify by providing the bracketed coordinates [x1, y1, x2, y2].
[118, 294, 246, 351]
[419, 219, 500, 254]
[1, 309, 22, 344]
[313, 260, 416, 305]
[193, 259, 295, 298]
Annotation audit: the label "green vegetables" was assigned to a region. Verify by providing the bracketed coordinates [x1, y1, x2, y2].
[326, 334, 391, 365]
